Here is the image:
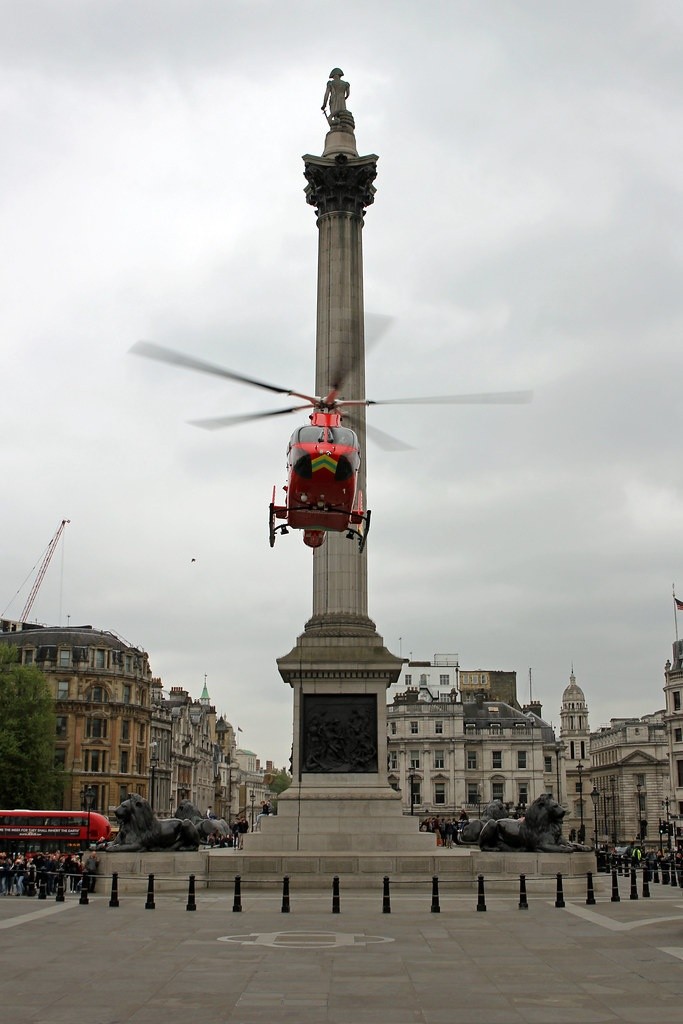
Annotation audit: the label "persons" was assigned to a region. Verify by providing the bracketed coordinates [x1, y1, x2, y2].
[321, 68, 350, 114]
[622, 841, 683, 870]
[503, 801, 528, 820]
[419, 810, 471, 848]
[0, 836, 108, 897]
[204, 800, 274, 849]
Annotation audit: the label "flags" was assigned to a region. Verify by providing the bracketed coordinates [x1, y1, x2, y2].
[675, 598, 683, 611]
[238, 727, 242, 732]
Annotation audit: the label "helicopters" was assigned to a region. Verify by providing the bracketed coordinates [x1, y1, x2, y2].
[134, 316, 533, 555]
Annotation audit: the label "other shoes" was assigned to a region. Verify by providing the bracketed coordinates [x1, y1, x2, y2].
[447, 846, 452, 849]
[1, 892, 21, 896]
[70, 891, 76, 893]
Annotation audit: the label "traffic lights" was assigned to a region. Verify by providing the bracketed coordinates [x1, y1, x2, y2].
[663, 821, 673, 836]
[677, 828, 683, 838]
[658, 825, 663, 835]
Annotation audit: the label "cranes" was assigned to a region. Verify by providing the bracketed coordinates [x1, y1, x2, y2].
[7, 516, 71, 622]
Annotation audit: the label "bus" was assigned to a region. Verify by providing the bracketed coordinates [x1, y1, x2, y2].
[0, 808, 111, 858]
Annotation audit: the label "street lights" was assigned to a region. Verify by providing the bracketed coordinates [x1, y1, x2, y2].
[251, 791, 256, 831]
[602, 787, 608, 840]
[85, 786, 94, 847]
[610, 778, 615, 846]
[150, 752, 159, 810]
[575, 760, 585, 843]
[590, 787, 601, 849]
[407, 765, 416, 814]
[476, 792, 481, 816]
[169, 795, 173, 816]
[637, 782, 642, 849]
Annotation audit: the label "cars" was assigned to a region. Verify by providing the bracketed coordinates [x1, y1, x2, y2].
[610, 852, 663, 865]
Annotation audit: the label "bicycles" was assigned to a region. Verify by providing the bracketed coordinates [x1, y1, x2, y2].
[55, 874, 68, 892]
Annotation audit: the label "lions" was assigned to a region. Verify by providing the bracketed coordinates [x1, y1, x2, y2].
[98, 792, 183, 852]
[460, 799, 509, 845]
[479, 791, 592, 854]
[174, 799, 232, 839]
